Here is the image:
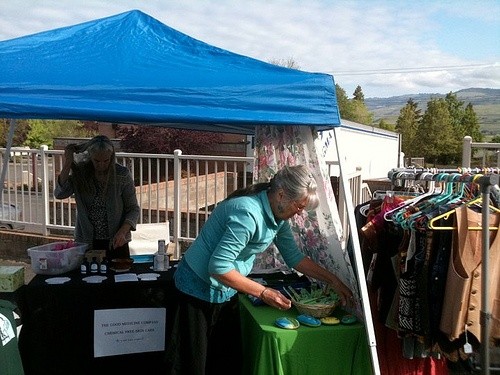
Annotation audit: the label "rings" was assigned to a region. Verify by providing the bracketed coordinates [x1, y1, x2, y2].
[279, 307, 282, 310]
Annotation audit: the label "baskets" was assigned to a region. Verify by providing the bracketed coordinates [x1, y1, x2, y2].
[291, 289, 340, 318]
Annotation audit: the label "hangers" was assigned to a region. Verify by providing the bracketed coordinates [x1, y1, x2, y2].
[360, 167, 500, 230]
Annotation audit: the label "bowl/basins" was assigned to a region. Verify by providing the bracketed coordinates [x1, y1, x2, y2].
[112, 258, 134, 270]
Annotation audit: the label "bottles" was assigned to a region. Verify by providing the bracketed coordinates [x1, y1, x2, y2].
[89, 256, 98, 275]
[100, 256, 108, 274]
[80, 256, 88, 276]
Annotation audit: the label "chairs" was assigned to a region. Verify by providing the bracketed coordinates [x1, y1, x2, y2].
[130, 221, 169, 254]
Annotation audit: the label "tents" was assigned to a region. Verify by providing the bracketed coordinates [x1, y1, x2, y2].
[0, 10, 381, 375]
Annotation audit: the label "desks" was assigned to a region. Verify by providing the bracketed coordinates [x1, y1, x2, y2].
[0, 257, 373, 375]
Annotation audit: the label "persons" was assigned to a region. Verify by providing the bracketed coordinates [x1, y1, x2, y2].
[54, 136, 140, 257]
[172, 165, 356, 375]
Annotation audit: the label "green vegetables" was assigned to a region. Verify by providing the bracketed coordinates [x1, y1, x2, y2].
[291, 282, 342, 306]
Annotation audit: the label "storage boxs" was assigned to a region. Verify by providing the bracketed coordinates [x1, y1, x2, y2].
[27, 241, 88, 275]
[0, 265, 25, 292]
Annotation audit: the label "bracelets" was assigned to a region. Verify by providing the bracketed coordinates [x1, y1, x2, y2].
[261, 287, 269, 301]
